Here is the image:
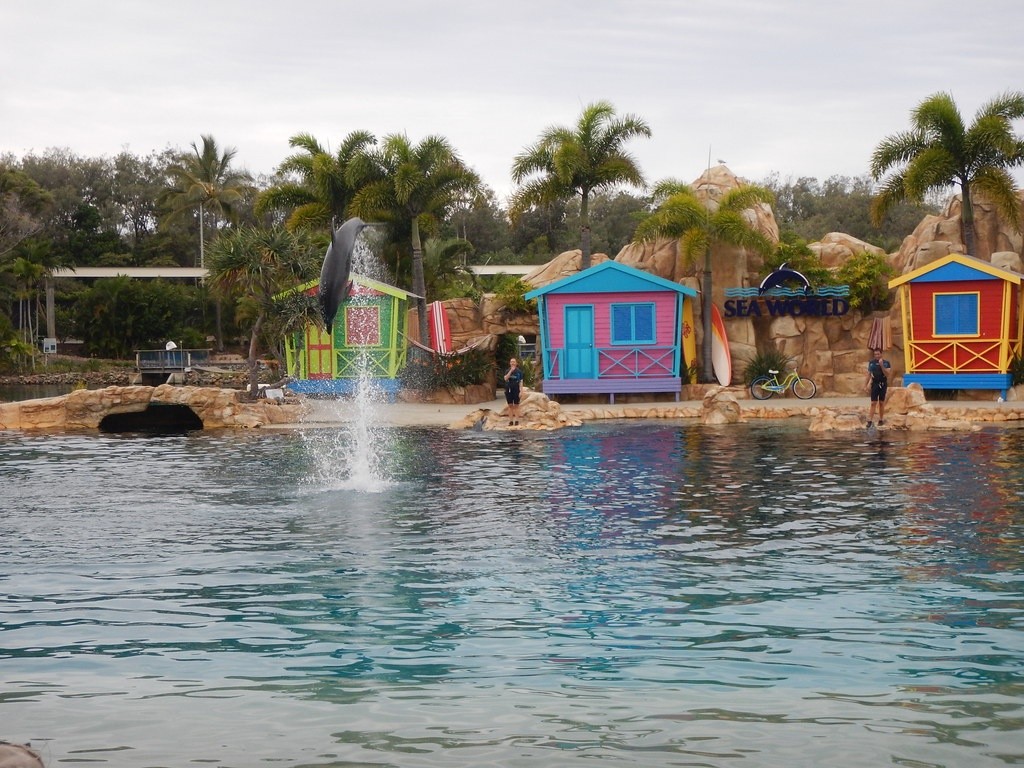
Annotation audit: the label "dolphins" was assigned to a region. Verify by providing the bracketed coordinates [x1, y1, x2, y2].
[319, 216, 387, 335]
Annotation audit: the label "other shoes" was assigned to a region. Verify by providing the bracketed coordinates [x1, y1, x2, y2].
[878, 420, 884, 426]
[515, 421, 518, 426]
[866, 421, 872, 427]
[509, 422, 513, 426]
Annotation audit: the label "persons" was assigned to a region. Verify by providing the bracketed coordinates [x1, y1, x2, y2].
[503, 358, 524, 426]
[863, 348, 890, 427]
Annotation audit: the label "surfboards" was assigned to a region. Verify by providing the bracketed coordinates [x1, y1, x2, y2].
[429, 300, 451, 353]
[711, 301, 731, 386]
[681, 296, 698, 383]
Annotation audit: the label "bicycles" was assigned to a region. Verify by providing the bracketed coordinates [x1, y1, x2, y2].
[750, 365, 817, 400]
